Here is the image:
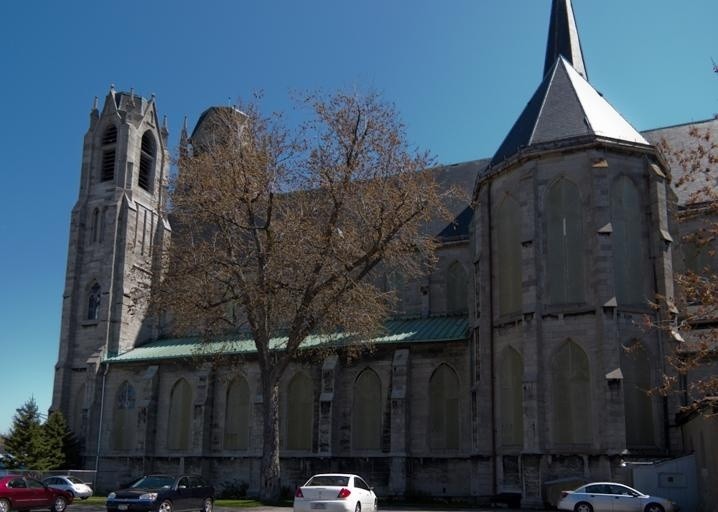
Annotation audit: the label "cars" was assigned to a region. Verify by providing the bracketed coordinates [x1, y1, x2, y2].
[555, 480, 678, 512]
[0, 452, 94, 512]
[105, 471, 215, 512]
[292, 471, 379, 512]
